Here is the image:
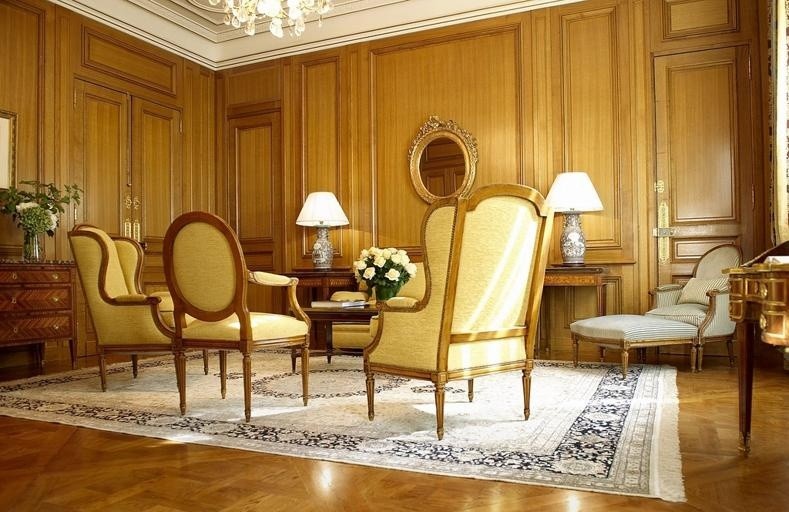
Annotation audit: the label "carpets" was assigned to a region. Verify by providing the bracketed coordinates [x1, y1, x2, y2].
[0, 348, 691, 504]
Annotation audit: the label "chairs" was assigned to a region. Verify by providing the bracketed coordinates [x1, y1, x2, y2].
[362, 183, 555, 441]
[162, 209, 312, 424]
[644, 243, 743, 372]
[67, 223, 210, 393]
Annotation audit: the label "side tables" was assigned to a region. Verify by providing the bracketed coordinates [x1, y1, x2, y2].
[544, 273, 625, 362]
[276, 269, 358, 349]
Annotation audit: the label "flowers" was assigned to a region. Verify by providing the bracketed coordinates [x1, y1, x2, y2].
[14, 201, 59, 257]
[353, 246, 417, 297]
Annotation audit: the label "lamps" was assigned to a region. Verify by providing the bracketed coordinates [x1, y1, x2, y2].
[209, 0, 335, 40]
[544, 171, 604, 264]
[294, 191, 350, 269]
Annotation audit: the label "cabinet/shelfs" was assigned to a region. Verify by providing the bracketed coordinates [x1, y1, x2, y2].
[0, 263, 79, 370]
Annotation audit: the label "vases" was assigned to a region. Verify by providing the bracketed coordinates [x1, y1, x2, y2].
[374, 285, 396, 303]
[22, 230, 47, 264]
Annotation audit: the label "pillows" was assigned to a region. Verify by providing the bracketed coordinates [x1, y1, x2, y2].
[675, 278, 728, 306]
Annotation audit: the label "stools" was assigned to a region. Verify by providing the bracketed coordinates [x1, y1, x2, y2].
[568, 313, 699, 380]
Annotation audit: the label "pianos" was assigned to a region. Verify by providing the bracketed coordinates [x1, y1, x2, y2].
[721, 237, 789, 455]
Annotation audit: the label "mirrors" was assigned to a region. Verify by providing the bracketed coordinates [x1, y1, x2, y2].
[0, 110, 20, 194]
[407, 115, 479, 206]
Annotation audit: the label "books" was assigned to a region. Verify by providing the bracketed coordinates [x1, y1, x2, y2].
[310, 301, 365, 308]
